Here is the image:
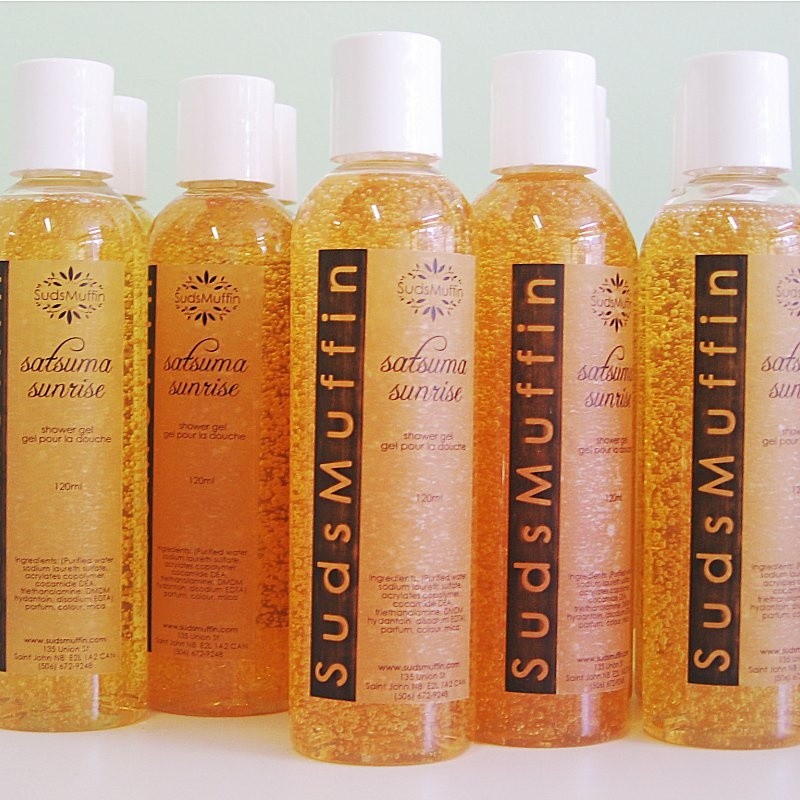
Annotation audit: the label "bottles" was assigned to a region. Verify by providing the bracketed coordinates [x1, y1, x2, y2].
[0, 29, 800, 767]
